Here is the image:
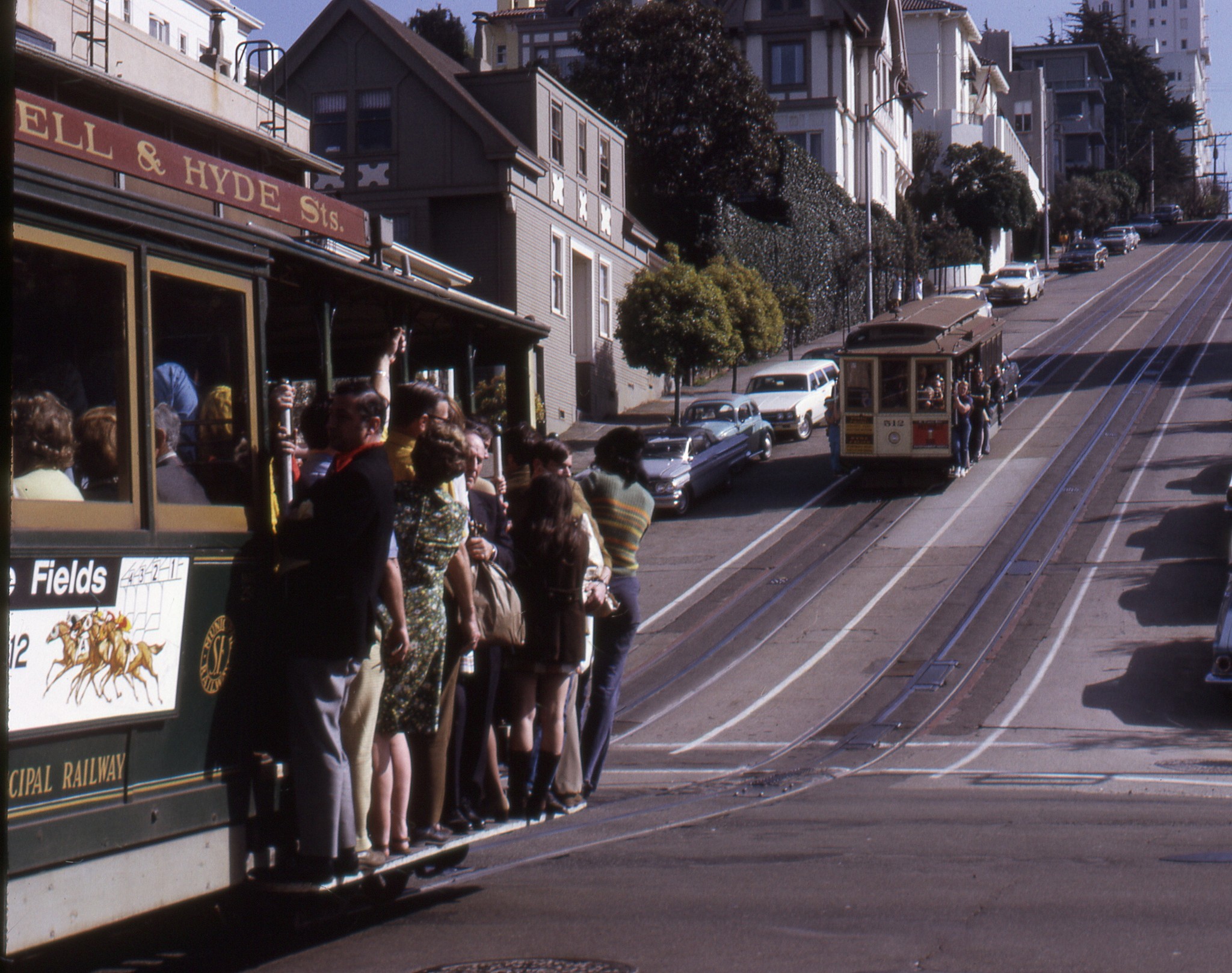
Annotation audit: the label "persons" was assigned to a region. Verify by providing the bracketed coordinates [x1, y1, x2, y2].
[1059, 224, 1069, 254]
[823, 352, 1005, 478]
[70, 405, 120, 507]
[9, 386, 86, 500]
[152, 325, 657, 895]
[1072, 226, 1083, 243]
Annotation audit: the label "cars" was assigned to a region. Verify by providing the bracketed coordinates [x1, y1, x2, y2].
[1127, 214, 1162, 238]
[1099, 229, 1137, 256]
[1059, 238, 1109, 274]
[801, 346, 845, 366]
[950, 286, 993, 322]
[1107, 225, 1141, 250]
[658, 392, 775, 464]
[1154, 203, 1183, 225]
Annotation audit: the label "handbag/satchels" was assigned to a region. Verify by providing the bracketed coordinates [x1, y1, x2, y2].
[460, 518, 527, 647]
[573, 511, 621, 617]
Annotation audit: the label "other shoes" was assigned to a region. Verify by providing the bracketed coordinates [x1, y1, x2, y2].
[942, 441, 999, 481]
[244, 779, 613, 889]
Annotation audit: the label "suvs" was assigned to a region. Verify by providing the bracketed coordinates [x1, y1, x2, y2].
[989, 260, 1046, 306]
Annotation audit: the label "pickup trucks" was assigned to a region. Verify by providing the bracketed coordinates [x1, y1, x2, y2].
[572, 425, 750, 517]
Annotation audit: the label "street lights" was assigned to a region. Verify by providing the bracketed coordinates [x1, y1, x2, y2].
[859, 90, 930, 323]
[1142, 126, 1177, 219]
[1035, 114, 1082, 266]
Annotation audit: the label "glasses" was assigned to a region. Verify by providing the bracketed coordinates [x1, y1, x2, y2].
[429, 412, 451, 425]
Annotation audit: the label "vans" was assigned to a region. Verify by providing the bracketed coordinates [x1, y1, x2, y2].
[742, 358, 841, 441]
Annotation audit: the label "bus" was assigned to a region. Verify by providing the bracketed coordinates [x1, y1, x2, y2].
[834, 286, 1009, 479]
[0, 87, 556, 876]
